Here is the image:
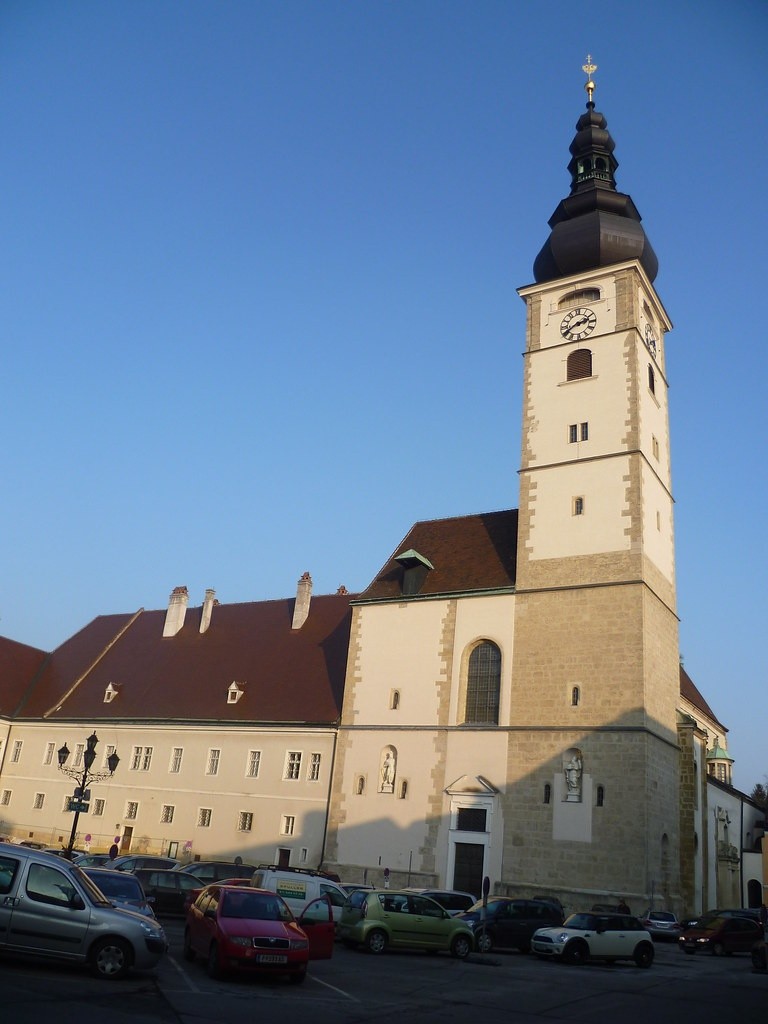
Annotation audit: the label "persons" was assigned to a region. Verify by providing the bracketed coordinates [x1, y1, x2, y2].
[616, 898, 631, 914]
[565, 756, 583, 793]
[760, 904, 768, 923]
[384, 751, 395, 784]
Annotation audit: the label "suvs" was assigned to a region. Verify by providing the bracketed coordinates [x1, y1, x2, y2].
[0, 843, 170, 980]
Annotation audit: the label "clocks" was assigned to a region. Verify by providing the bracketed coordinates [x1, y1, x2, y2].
[645, 323, 657, 359]
[561, 307, 598, 342]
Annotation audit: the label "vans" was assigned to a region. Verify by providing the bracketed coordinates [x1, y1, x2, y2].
[251, 864, 349, 923]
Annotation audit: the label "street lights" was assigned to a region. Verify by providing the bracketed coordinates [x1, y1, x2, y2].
[57, 730, 120, 860]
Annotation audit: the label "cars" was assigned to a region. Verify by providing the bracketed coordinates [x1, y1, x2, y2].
[183, 878, 251, 913]
[696, 910, 764, 931]
[340, 888, 476, 958]
[186, 886, 338, 985]
[378, 890, 478, 919]
[530, 912, 655, 969]
[322, 882, 385, 907]
[128, 868, 206, 912]
[679, 917, 765, 956]
[533, 895, 566, 912]
[644, 911, 680, 943]
[455, 897, 565, 955]
[0, 836, 181, 873]
[177, 860, 257, 886]
[752, 941, 768, 971]
[591, 904, 631, 916]
